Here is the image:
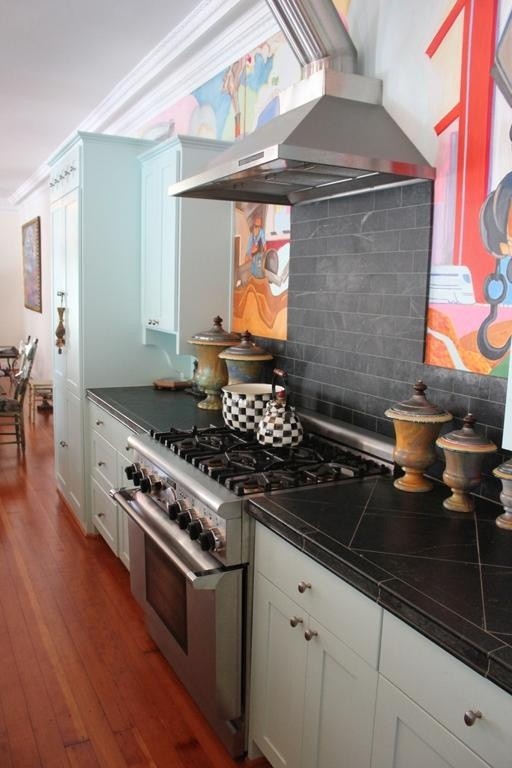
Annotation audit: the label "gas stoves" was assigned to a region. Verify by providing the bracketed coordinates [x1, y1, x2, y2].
[148, 422, 391, 498]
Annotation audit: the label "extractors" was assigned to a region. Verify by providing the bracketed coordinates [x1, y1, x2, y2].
[162, 1, 437, 207]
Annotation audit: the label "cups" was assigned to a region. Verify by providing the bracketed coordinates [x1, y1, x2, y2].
[218, 328, 274, 388]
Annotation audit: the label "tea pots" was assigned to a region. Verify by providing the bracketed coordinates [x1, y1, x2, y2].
[255, 367, 304, 450]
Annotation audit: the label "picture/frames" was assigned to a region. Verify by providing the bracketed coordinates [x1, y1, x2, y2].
[21, 216, 42, 313]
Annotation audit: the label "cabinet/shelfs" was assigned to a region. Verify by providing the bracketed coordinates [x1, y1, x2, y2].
[86, 399, 136, 574]
[371, 608, 511, 768]
[45, 131, 159, 537]
[134, 134, 238, 359]
[248, 520, 382, 768]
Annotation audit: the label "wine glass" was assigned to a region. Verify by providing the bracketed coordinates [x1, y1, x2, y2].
[435, 412, 499, 514]
[492, 456, 512, 531]
[384, 379, 453, 493]
[185, 315, 229, 411]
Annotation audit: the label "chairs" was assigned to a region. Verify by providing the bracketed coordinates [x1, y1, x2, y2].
[1, 334, 39, 454]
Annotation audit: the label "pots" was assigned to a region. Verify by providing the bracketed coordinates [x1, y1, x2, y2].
[220, 382, 287, 433]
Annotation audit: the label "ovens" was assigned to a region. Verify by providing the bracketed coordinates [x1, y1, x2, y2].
[109, 439, 245, 721]
